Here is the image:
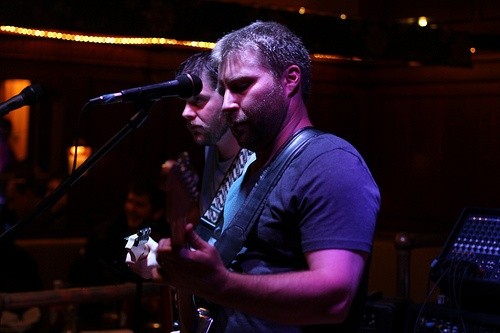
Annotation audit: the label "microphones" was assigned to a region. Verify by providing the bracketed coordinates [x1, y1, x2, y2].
[90, 73, 203, 106]
[0, 84, 43, 117]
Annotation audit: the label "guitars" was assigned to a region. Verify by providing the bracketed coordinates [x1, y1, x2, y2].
[123, 225, 153, 262]
[164, 240, 218, 331]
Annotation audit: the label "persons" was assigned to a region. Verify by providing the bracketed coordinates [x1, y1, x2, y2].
[125, 50, 240, 333]
[84, 184, 173, 328]
[156, 23, 380, 333]
